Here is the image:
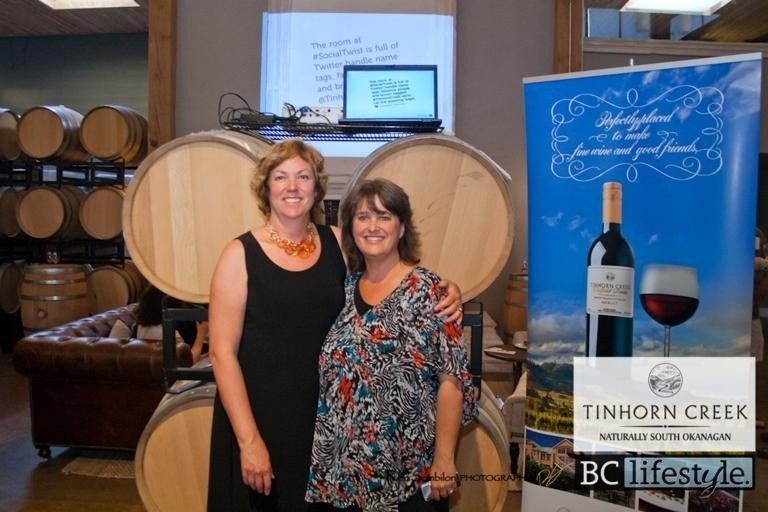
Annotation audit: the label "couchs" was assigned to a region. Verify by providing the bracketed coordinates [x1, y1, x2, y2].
[14, 300, 194, 463]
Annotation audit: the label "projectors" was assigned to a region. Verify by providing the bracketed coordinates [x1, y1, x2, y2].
[282, 106, 343, 133]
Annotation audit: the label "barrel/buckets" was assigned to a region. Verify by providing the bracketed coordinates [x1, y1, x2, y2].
[134, 358, 218, 511]
[121, 129, 326, 305]
[0, 105, 147, 169]
[0, 186, 126, 239]
[450, 379, 511, 511]
[0, 262, 148, 338]
[338, 133, 517, 301]
[502, 273, 527, 344]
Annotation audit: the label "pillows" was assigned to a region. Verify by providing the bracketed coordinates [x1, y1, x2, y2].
[108, 318, 131, 338]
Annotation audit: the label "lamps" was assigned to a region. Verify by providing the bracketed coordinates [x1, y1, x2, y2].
[618, 0, 733, 18]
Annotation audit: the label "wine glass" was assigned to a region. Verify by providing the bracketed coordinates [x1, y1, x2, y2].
[639, 264, 700, 356]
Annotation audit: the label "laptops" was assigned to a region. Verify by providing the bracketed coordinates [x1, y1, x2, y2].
[338, 65, 442, 137]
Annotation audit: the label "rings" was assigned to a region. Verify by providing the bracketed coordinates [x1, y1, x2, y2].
[457, 305, 464, 312]
[448, 489, 454, 494]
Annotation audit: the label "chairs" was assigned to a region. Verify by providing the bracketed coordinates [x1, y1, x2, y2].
[502, 369, 528, 495]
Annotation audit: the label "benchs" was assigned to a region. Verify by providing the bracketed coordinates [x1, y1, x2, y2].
[462, 309, 515, 402]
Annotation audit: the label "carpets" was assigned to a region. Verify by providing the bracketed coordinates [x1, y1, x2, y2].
[62, 455, 136, 480]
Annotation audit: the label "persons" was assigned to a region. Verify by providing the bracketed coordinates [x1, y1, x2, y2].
[299, 178, 474, 511]
[205, 138, 464, 511]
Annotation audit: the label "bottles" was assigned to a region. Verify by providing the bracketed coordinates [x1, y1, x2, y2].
[583, 180, 636, 360]
[519, 260, 528, 271]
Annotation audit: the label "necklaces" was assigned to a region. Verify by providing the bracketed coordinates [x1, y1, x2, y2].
[264, 220, 317, 260]
